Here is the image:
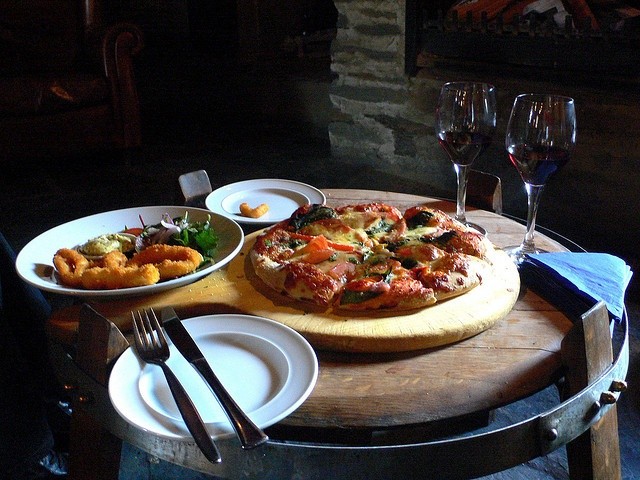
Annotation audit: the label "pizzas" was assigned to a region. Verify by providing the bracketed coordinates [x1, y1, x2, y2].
[249, 201, 496, 311]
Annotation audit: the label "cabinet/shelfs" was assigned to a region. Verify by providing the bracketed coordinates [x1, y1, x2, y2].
[405, 0, 640, 90]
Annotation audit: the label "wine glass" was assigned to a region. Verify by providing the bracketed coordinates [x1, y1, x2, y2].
[505, 94, 576, 266]
[435, 81, 497, 237]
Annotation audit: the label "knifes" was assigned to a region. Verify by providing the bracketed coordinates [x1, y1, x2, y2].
[161, 306, 269, 450]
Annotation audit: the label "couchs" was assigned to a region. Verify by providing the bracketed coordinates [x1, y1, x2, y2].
[0, 0, 145, 164]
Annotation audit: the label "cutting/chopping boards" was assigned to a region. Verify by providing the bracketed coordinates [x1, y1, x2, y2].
[47, 216, 520, 354]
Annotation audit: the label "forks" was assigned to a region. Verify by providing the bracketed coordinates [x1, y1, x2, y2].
[130, 307, 222, 464]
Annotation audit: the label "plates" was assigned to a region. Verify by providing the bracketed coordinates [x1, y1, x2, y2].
[15, 206, 244, 296]
[108, 314, 319, 440]
[205, 178, 326, 225]
